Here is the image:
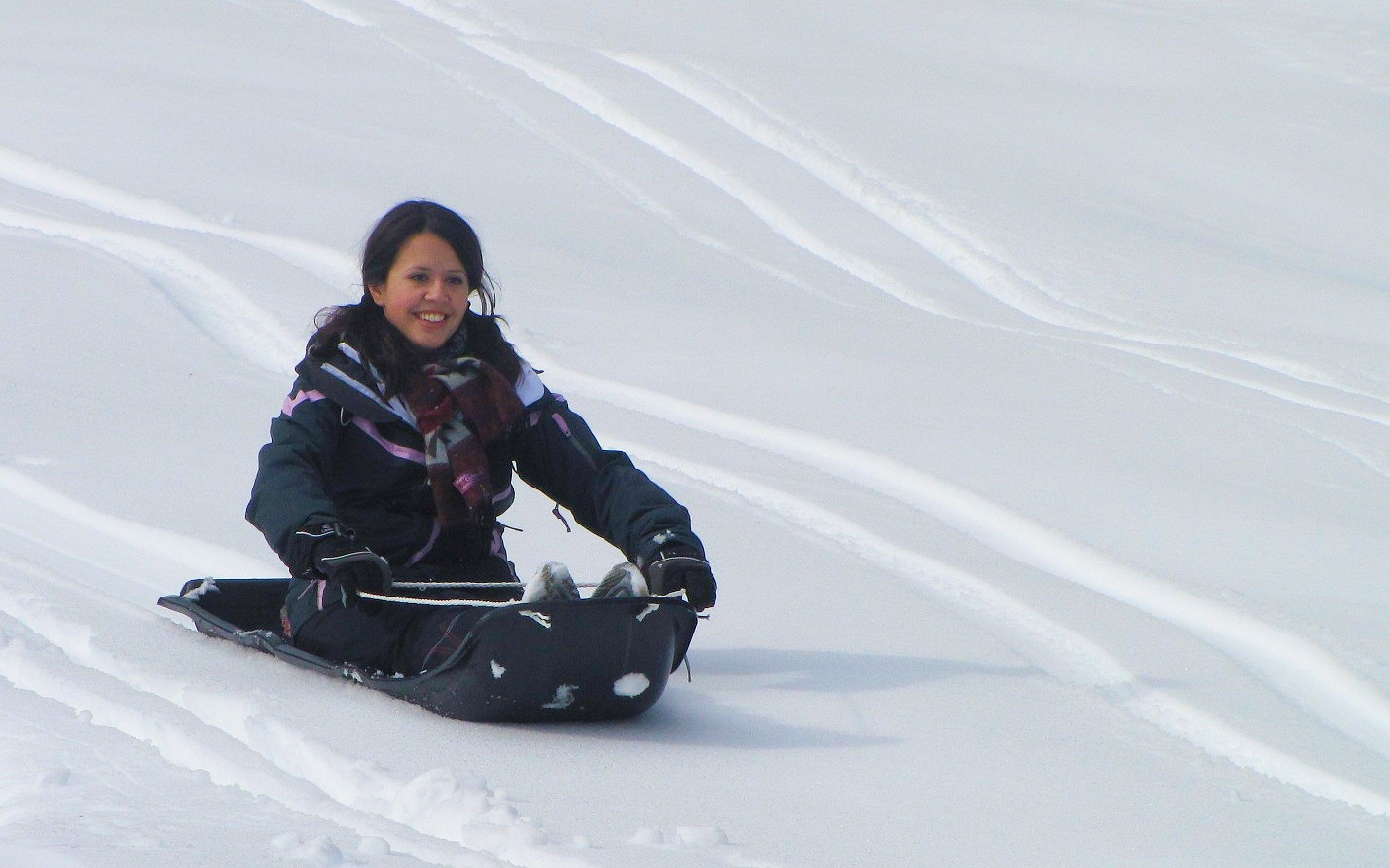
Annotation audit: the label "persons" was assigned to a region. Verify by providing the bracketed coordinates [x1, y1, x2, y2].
[247, 200, 718, 676]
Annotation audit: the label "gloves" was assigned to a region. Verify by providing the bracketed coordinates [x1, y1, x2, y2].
[647, 547, 717, 611]
[313, 537, 392, 614]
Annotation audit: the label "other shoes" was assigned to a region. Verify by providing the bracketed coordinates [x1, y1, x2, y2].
[595, 563, 648, 600]
[542, 563, 582, 602]
